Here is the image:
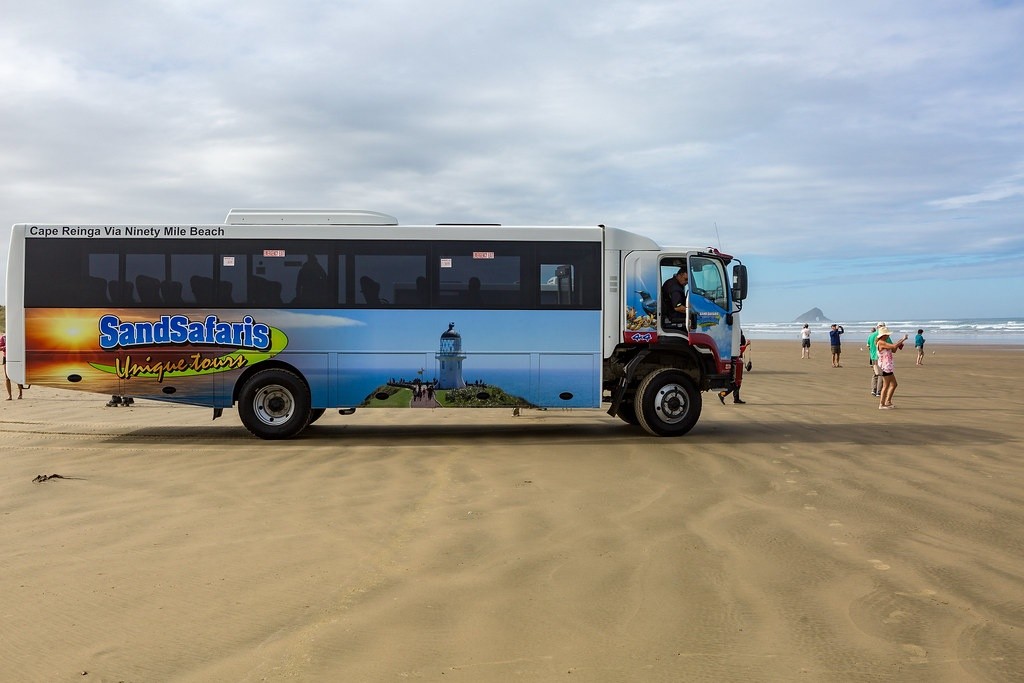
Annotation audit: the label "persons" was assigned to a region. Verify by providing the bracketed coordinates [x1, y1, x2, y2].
[661, 266, 688, 318]
[829, 324, 845, 368]
[0, 333, 23, 401]
[914, 329, 926, 366]
[718, 328, 751, 406]
[867, 321, 908, 410]
[801, 323, 811, 359]
[105, 395, 135, 407]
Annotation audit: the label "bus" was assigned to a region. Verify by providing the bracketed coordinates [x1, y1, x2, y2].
[4, 207, 749, 440]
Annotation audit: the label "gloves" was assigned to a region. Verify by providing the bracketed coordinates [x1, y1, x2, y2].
[836, 325, 839, 329]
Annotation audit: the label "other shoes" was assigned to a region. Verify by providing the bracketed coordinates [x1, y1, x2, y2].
[734, 399, 746, 404]
[718, 393, 725, 405]
[879, 404, 896, 409]
[871, 391, 881, 397]
[832, 364, 836, 368]
[837, 365, 842, 368]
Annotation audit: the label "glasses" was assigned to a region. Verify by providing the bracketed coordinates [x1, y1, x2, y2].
[680, 274, 688, 281]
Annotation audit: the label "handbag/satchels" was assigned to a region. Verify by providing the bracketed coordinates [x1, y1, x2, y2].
[745, 361, 752, 371]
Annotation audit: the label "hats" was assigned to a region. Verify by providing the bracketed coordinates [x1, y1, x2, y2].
[831, 324, 836, 328]
[877, 327, 893, 338]
[876, 322, 885, 328]
